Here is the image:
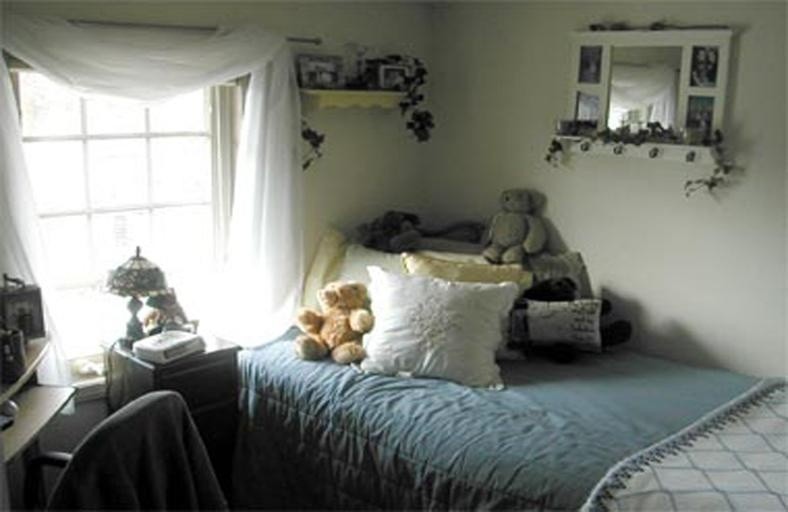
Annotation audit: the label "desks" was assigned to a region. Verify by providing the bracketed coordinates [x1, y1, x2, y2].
[0, 335, 76, 512]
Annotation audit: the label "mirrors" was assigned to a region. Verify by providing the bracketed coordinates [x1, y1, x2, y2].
[553, 30, 731, 150]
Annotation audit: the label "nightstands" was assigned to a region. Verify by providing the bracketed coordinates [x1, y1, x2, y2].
[104, 335, 239, 501]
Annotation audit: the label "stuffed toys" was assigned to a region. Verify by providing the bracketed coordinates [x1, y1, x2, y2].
[484, 188, 546, 265]
[293, 281, 374, 365]
[511, 277, 633, 365]
[363, 209, 421, 258]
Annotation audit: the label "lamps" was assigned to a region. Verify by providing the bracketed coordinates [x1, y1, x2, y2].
[105, 246, 167, 348]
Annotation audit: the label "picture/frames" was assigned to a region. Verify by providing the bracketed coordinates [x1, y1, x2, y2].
[299, 53, 345, 90]
[378, 66, 409, 89]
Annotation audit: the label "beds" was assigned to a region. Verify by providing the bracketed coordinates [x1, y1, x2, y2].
[232, 328, 788, 512]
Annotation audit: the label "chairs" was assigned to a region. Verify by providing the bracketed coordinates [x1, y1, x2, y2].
[22, 392, 227, 512]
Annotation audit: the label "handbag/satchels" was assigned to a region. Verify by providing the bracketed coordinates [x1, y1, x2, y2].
[1, 325, 26, 384]
